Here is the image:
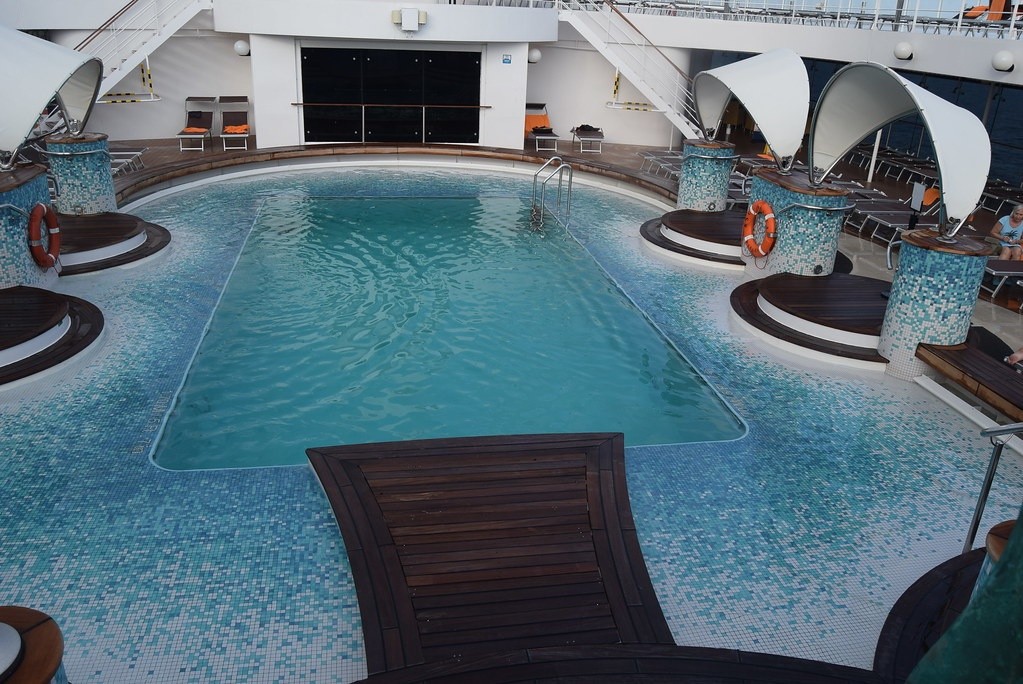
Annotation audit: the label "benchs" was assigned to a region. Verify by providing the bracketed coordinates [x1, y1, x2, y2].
[917, 343, 1023, 424]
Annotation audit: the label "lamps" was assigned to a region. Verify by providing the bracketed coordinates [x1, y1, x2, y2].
[528, 49, 542, 63]
[991, 49, 1014, 72]
[233, 39, 250, 57]
[894, 41, 913, 60]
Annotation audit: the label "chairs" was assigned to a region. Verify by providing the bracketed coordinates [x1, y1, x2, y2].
[218, 95, 250, 151]
[175, 97, 217, 150]
[638, 143, 1023, 315]
[949, 0, 1023, 39]
[571, 125, 605, 154]
[13, 122, 150, 196]
[526, 101, 561, 152]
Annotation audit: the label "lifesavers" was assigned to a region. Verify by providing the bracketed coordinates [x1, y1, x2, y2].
[27, 202, 61, 268]
[740, 200, 779, 259]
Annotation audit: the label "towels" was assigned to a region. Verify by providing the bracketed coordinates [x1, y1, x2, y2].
[223, 123, 250, 134]
[183, 126, 209, 133]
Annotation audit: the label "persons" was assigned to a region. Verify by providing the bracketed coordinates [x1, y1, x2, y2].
[991, 205, 1023, 285]
